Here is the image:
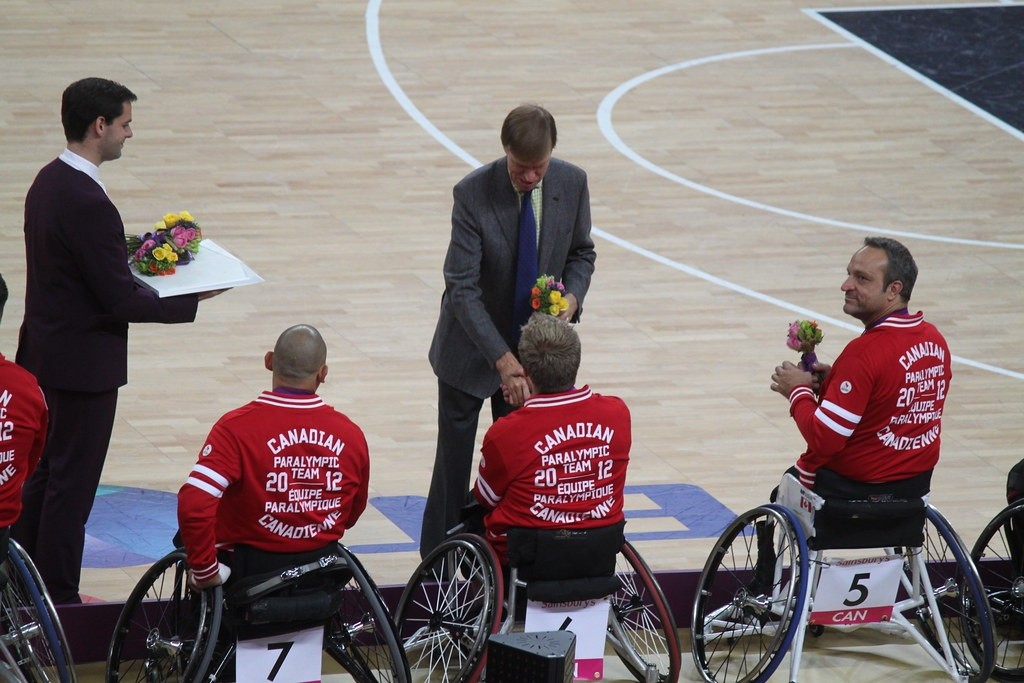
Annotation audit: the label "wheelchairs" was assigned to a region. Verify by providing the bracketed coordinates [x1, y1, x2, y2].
[105, 542, 412, 683]
[690, 474, 1024, 683]
[0, 527, 76, 683]
[394, 521, 682, 683]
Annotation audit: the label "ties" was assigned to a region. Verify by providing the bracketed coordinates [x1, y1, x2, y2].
[515, 191, 538, 322]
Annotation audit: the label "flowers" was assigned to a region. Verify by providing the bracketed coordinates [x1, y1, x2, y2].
[786, 318, 825, 398]
[529, 274, 569, 317]
[124, 210, 203, 259]
[128, 240, 196, 276]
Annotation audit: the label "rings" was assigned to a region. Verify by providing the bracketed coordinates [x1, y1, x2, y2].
[566, 317, 571, 322]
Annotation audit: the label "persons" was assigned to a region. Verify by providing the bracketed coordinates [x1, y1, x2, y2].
[13, 77, 234, 607]
[176, 325, 371, 597]
[0, 272, 49, 565]
[474, 311, 631, 601]
[420, 105, 597, 581]
[770, 236, 953, 501]
